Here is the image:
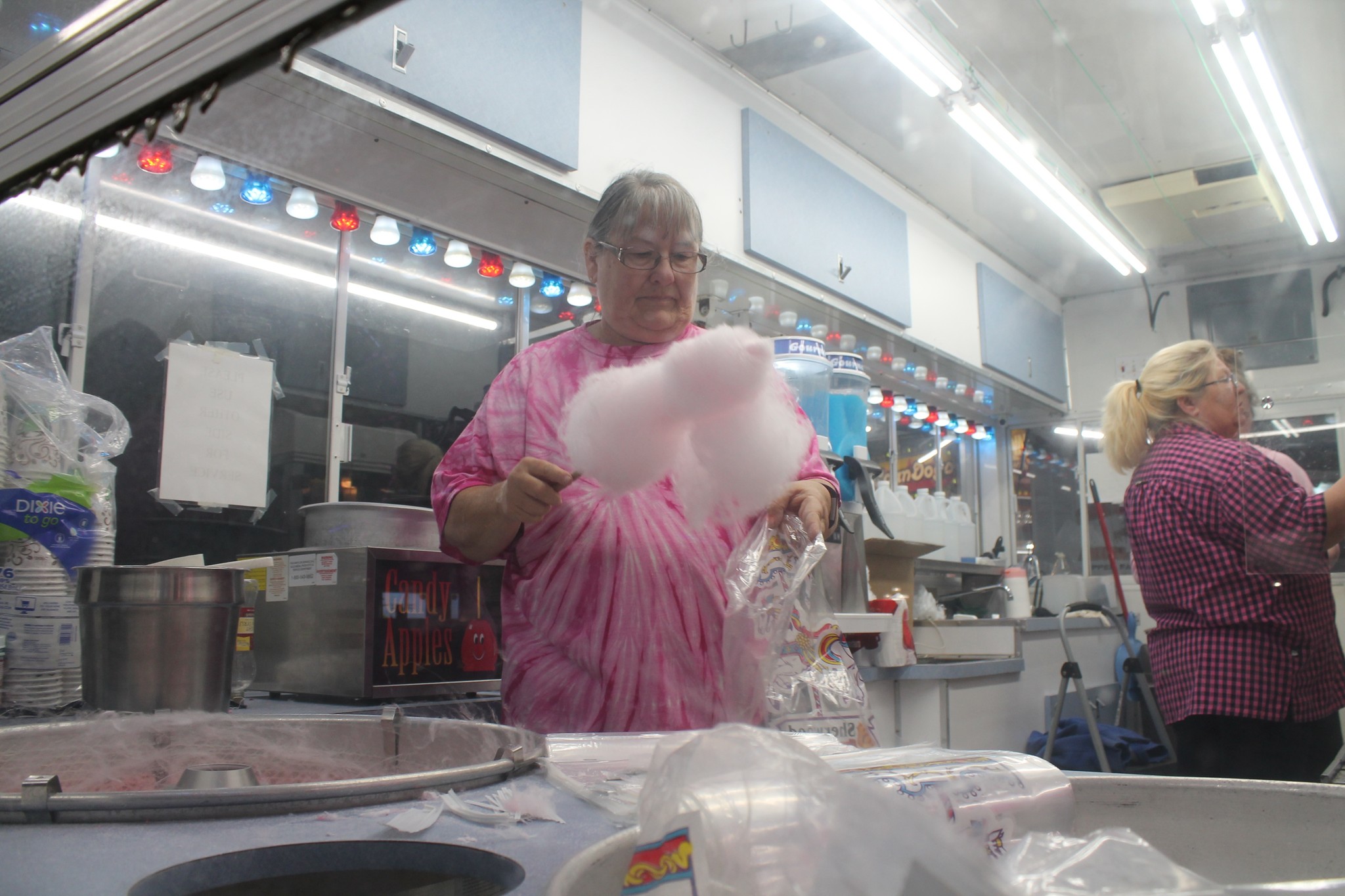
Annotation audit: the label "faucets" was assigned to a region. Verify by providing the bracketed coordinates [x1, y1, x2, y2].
[938, 583, 1016, 605]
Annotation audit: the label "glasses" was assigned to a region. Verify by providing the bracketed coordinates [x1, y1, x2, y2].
[1198, 372, 1238, 387]
[598, 240, 708, 274]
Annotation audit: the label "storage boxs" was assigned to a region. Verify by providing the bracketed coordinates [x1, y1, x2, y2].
[865, 538, 946, 633]
[0, 363, 116, 711]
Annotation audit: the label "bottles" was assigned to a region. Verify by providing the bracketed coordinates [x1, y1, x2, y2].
[863, 480, 977, 563]
[231, 579, 258, 706]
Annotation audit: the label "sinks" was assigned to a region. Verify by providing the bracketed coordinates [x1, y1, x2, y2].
[903, 653, 993, 666]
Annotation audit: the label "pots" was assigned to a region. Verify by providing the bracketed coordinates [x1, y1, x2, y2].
[70, 564, 251, 715]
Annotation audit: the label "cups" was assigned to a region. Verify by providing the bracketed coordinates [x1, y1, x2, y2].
[0, 387, 116, 711]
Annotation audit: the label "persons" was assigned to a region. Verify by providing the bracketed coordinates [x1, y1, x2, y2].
[388, 438, 443, 511]
[1102, 337, 1345, 789]
[1210, 343, 1318, 502]
[430, 171, 846, 738]
